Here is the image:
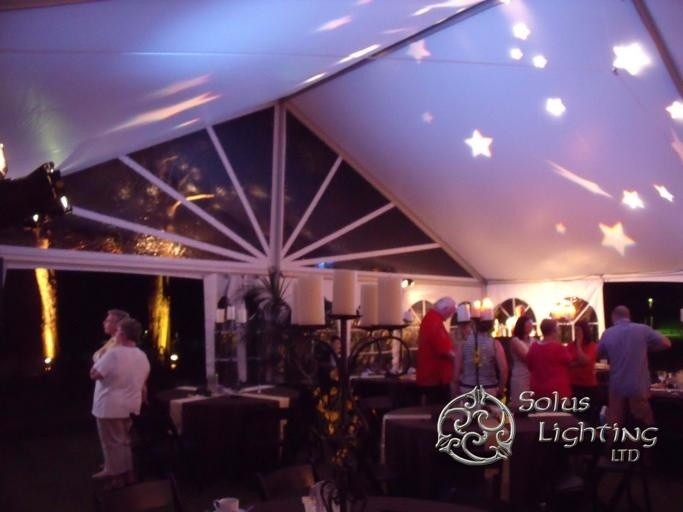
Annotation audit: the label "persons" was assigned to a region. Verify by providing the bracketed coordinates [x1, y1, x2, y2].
[88, 318, 151, 492]
[508, 313, 539, 413]
[328, 334, 341, 354]
[458, 318, 509, 407]
[466, 316, 479, 334]
[449, 322, 470, 356]
[412, 296, 460, 407]
[595, 305, 672, 476]
[89, 309, 133, 480]
[522, 318, 577, 408]
[560, 317, 600, 455]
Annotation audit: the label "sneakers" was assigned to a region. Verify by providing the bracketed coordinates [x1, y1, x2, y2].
[104, 470, 138, 493]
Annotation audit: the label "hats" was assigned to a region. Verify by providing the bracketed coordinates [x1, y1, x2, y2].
[456, 303, 471, 323]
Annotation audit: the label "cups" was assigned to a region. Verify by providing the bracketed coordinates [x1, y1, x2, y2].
[206, 373, 219, 393]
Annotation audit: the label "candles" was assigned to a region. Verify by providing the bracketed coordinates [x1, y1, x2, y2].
[288, 268, 404, 327]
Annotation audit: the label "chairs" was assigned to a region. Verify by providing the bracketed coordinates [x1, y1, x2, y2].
[92, 473, 189, 511]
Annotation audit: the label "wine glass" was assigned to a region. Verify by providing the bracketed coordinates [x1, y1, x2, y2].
[658, 370, 682, 392]
